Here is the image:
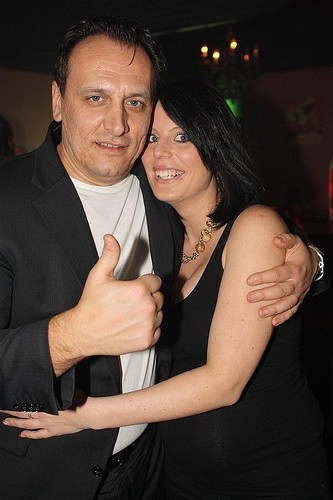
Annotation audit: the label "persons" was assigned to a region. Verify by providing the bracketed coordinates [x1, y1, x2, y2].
[1, 114, 28, 165]
[0, 79, 319, 500]
[0, 17, 332, 499]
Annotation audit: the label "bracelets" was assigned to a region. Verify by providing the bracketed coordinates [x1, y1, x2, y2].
[306, 243, 328, 284]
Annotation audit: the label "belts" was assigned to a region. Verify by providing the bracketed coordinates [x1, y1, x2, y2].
[107, 423, 153, 469]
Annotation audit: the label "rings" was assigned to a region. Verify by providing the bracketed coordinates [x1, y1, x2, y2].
[27, 412, 33, 419]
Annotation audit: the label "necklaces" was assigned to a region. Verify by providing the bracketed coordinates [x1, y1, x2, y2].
[181, 207, 217, 263]
